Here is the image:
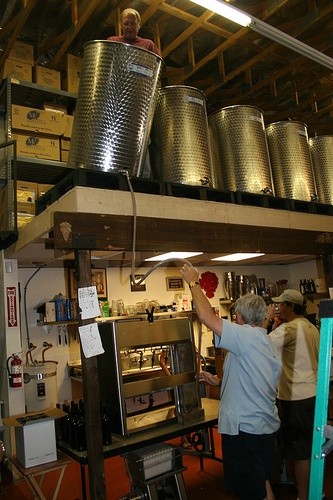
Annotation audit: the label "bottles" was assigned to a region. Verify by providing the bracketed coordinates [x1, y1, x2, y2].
[137, 299, 188, 313]
[299, 279, 316, 294]
[56, 399, 111, 451]
[126, 305, 134, 315]
[103, 299, 124, 317]
[268, 281, 275, 297]
[224, 271, 257, 300]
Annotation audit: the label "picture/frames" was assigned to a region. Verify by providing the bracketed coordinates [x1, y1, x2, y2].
[68, 267, 108, 299]
[166, 277, 185, 290]
[130, 275, 146, 291]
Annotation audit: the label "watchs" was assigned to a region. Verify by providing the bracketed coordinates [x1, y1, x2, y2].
[189, 281, 199, 290]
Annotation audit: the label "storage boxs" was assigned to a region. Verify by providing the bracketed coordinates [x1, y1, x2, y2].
[0, 39, 85, 231]
[15, 420, 57, 468]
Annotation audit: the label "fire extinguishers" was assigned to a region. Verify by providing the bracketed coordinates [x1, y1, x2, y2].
[6, 351, 23, 388]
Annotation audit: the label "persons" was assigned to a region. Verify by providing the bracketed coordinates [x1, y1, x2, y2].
[181, 259, 298, 500]
[107, 8, 163, 58]
[266, 289, 320, 500]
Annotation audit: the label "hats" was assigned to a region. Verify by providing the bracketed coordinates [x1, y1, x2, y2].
[271, 289, 304, 306]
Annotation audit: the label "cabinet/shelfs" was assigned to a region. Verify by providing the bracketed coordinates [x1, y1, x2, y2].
[82, 316, 206, 438]
[0, 77, 77, 235]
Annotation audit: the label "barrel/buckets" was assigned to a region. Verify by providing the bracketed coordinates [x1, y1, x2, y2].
[67, 40, 166, 178]
[147, 85, 214, 187]
[208, 105, 275, 196]
[266, 122, 318, 203]
[308, 135, 333, 204]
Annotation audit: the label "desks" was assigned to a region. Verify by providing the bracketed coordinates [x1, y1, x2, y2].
[9, 450, 71, 500]
[56, 397, 223, 500]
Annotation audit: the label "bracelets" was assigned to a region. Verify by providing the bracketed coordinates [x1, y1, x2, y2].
[264, 317, 272, 323]
